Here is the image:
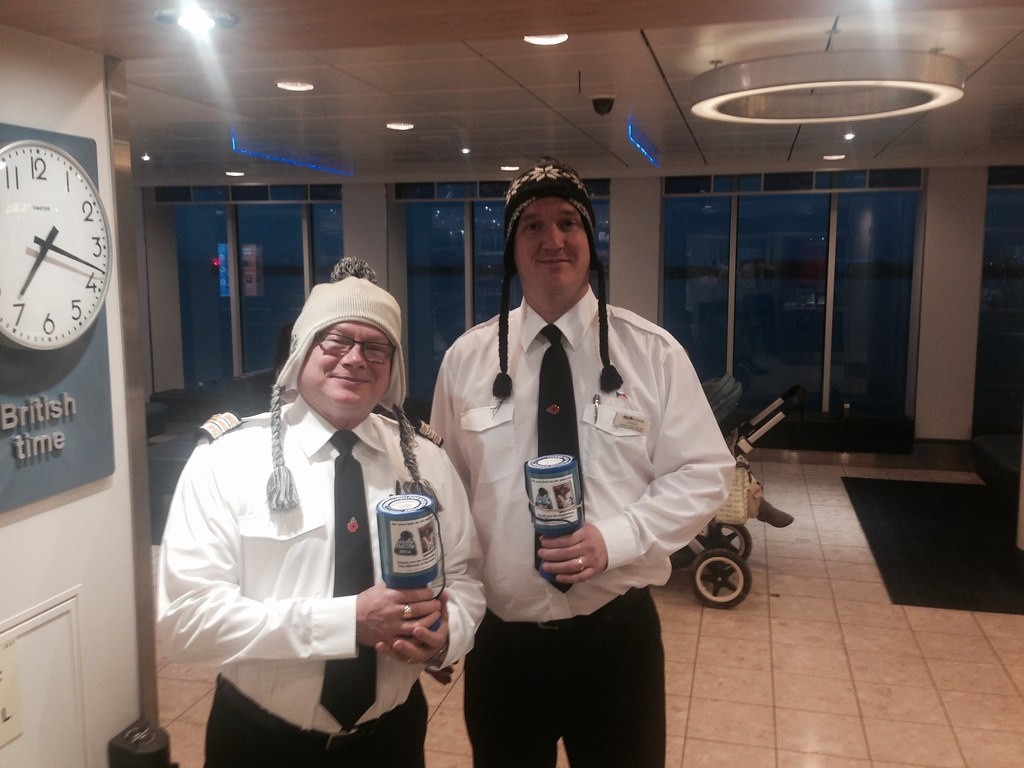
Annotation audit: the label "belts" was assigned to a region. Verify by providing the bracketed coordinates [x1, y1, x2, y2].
[214, 676, 420, 750]
[510, 585, 650, 630]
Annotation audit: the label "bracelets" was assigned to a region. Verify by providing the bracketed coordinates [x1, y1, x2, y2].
[424, 644, 447, 666]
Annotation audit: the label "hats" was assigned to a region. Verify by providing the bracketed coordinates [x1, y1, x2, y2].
[493, 161, 624, 418]
[267, 257, 445, 513]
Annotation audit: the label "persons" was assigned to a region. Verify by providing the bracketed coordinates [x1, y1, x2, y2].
[156, 259, 488, 768]
[424, 156, 737, 767]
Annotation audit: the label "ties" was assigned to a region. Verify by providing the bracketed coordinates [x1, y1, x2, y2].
[319, 429, 378, 732]
[533, 324, 586, 595]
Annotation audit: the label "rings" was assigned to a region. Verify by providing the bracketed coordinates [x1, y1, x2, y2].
[577, 558, 584, 571]
[407, 658, 416, 664]
[403, 606, 412, 620]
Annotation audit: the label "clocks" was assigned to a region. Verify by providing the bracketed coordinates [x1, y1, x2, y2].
[0, 138, 113, 352]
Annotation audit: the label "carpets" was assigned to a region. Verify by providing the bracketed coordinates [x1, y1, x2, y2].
[839, 475, 1024, 615]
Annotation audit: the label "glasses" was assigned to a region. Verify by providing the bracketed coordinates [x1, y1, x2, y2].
[314, 331, 395, 365]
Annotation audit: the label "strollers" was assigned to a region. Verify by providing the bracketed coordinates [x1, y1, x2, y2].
[670, 371, 807, 608]
